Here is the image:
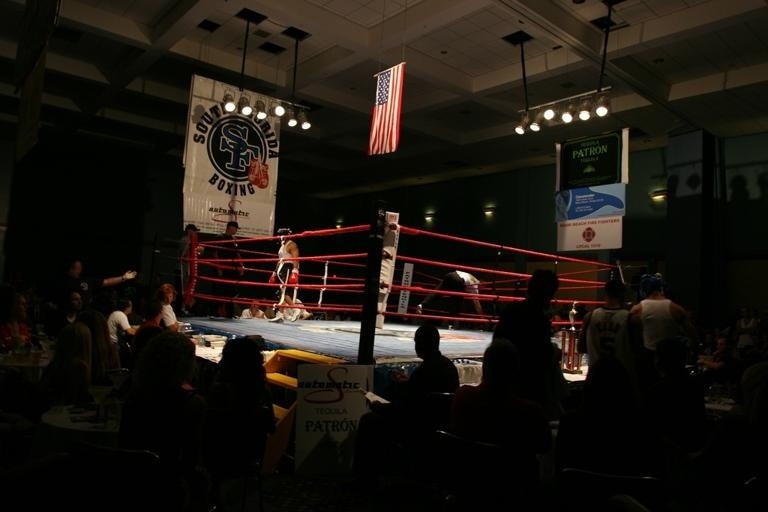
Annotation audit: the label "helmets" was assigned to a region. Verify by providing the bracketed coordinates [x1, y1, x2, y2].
[277, 229, 293, 235]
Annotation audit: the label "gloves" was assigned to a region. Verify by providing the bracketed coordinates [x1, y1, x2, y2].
[288, 273, 300, 284]
[269, 271, 278, 283]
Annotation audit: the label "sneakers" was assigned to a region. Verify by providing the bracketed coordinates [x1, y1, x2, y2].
[269, 311, 285, 322]
[291, 308, 300, 322]
[449, 325, 455, 331]
[416, 304, 422, 314]
[302, 311, 313, 319]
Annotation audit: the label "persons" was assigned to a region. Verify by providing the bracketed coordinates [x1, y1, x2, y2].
[268, 227, 300, 323]
[209, 222, 246, 320]
[1, 268, 767, 512]
[166, 223, 201, 315]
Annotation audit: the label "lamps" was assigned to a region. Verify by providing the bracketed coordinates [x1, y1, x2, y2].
[223, 8, 312, 131]
[502, 0, 623, 135]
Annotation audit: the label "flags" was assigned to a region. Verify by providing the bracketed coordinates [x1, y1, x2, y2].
[367, 61, 404, 155]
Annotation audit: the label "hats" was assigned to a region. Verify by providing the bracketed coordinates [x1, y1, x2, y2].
[186, 224, 200, 232]
[227, 221, 240, 229]
